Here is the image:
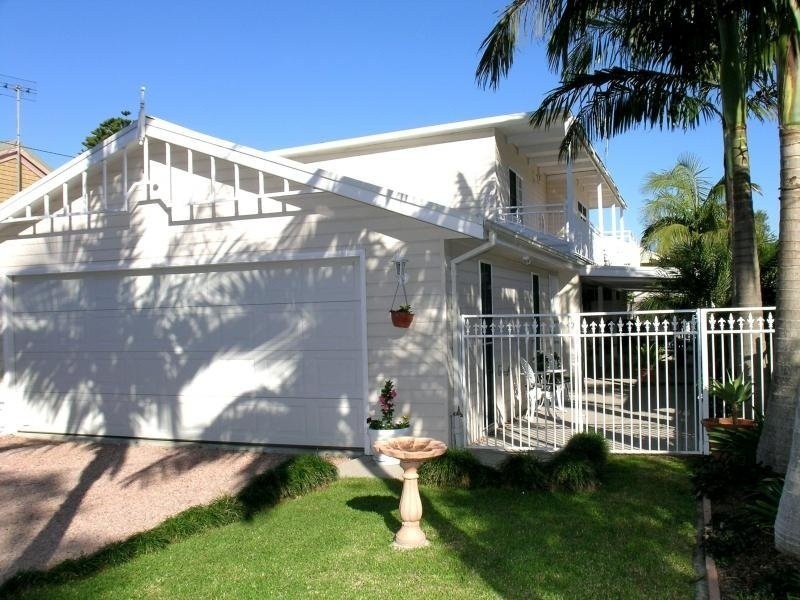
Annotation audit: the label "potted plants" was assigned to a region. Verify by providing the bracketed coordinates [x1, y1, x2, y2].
[636, 342, 665, 385]
[389, 305, 415, 329]
[701, 369, 758, 460]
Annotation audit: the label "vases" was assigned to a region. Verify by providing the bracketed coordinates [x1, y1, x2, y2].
[367, 428, 411, 461]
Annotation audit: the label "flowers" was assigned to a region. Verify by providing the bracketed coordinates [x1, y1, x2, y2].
[367, 380, 411, 430]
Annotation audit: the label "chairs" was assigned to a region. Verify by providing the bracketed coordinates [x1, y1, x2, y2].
[520, 356, 560, 423]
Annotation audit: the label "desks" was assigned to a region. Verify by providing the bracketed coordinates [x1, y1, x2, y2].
[520, 369, 567, 419]
